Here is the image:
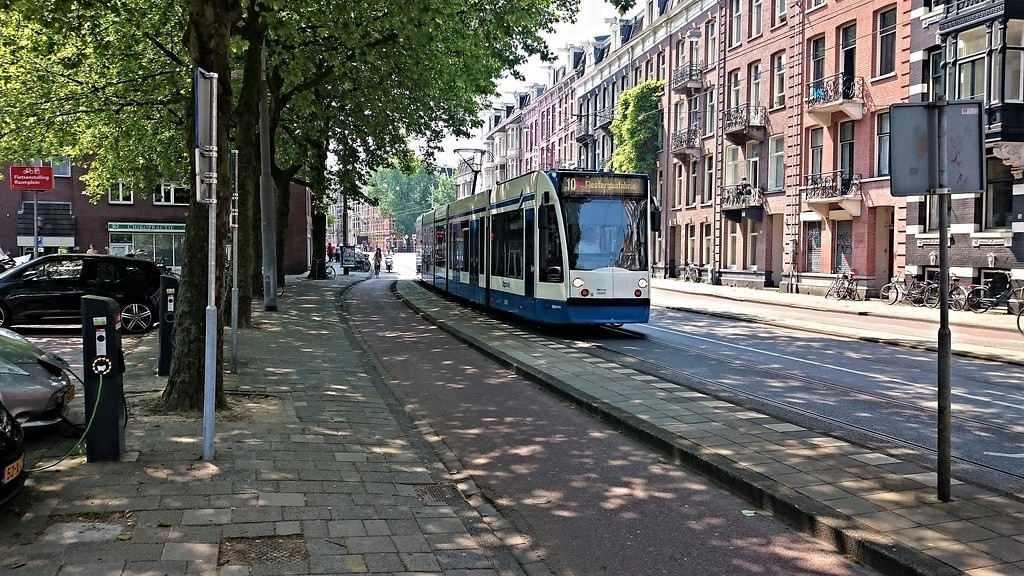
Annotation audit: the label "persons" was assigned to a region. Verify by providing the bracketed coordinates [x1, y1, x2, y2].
[87, 243, 97, 254]
[327, 243, 393, 274]
[574, 228, 601, 253]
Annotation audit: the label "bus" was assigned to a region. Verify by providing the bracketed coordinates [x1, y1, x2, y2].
[416, 148, 664, 331]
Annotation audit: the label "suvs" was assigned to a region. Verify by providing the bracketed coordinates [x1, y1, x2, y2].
[0, 253, 172, 335]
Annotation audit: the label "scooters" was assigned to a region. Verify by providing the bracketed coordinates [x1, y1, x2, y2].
[385, 255, 393, 272]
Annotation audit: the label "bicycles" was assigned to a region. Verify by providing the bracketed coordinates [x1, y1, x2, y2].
[325, 261, 337, 278]
[879, 267, 968, 312]
[373, 259, 381, 279]
[683, 263, 700, 282]
[824, 265, 860, 301]
[966, 272, 1024, 316]
[1016, 308, 1024, 335]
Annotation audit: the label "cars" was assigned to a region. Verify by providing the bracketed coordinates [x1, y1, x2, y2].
[0, 329, 75, 431]
[0, 248, 16, 272]
[353, 252, 371, 270]
